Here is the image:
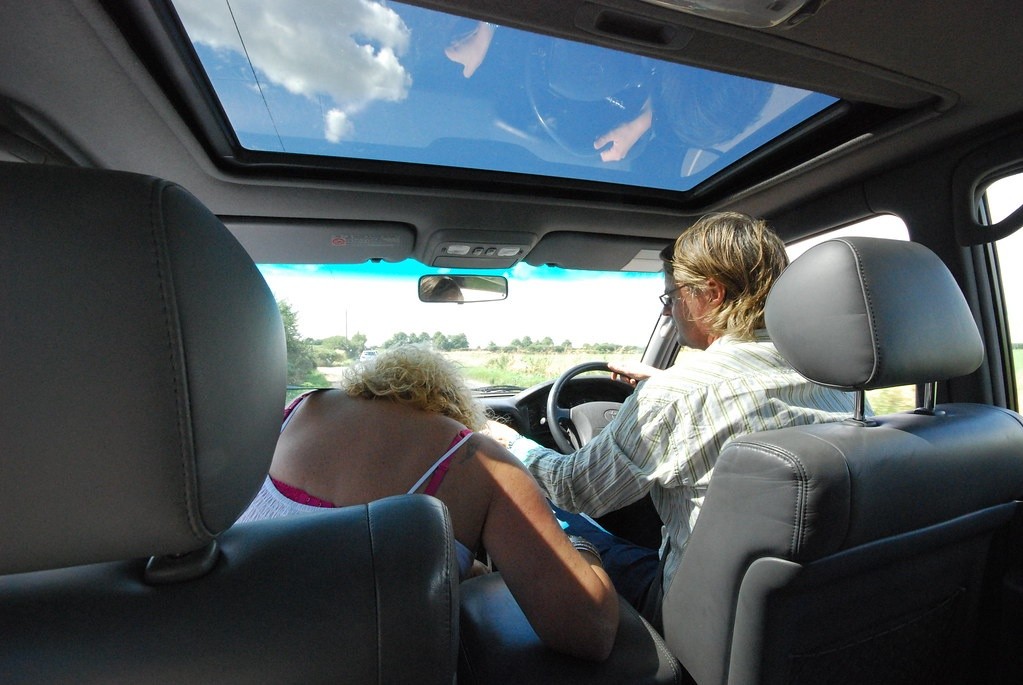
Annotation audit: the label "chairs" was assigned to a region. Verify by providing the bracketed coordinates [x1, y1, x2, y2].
[0, 158, 462, 685]
[663, 236, 1023, 684]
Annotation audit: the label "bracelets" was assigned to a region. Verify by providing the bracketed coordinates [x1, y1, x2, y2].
[573, 541, 603, 565]
[485, 22, 499, 28]
[508, 434, 524, 449]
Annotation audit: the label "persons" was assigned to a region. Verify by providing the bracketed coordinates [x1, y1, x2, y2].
[231, 344, 621, 663]
[479, 212, 875, 639]
[445, 19, 774, 162]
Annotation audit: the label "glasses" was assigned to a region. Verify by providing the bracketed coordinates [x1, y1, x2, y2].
[659, 283, 689, 308]
